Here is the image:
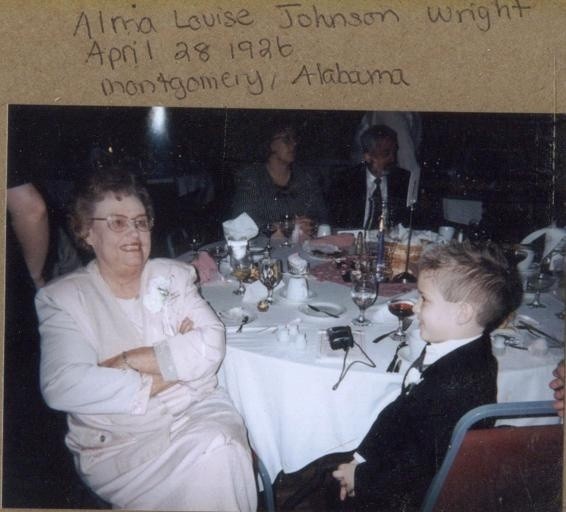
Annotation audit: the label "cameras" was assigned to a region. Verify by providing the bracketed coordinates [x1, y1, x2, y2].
[327, 325, 354, 350]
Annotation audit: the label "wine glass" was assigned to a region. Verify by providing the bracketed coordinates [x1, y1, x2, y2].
[229, 251, 253, 297]
[281, 214, 294, 247]
[387, 298, 417, 340]
[258, 258, 282, 303]
[261, 226, 277, 251]
[351, 274, 378, 325]
[526, 272, 557, 309]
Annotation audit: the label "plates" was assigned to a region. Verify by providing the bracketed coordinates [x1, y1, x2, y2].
[297, 300, 348, 320]
[513, 313, 541, 331]
[303, 243, 347, 261]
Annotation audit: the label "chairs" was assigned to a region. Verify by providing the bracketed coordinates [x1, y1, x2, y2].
[419, 400, 562, 511]
[252, 449, 276, 512]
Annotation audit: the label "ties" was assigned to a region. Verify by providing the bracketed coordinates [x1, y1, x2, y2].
[365, 178, 383, 230]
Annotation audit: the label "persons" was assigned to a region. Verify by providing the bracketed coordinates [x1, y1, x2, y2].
[352, 109, 426, 230]
[259, 234, 524, 512]
[546, 358, 566, 425]
[325, 124, 411, 232]
[29, 172, 259, 511]
[6, 137, 50, 476]
[227, 122, 331, 234]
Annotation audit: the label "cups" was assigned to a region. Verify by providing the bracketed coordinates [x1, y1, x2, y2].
[227, 237, 248, 262]
[439, 226, 455, 241]
[316, 224, 331, 238]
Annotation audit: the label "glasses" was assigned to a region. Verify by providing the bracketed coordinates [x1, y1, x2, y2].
[93, 213, 154, 231]
[271, 134, 298, 143]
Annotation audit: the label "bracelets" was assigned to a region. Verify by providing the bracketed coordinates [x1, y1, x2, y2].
[122, 352, 141, 371]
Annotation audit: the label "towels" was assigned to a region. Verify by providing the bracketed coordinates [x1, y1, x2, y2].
[222, 211, 258, 257]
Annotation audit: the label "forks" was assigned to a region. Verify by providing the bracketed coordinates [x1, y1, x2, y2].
[373, 317, 414, 344]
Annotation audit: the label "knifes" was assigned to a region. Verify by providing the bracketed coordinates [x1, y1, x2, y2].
[233, 313, 249, 335]
[307, 303, 342, 318]
